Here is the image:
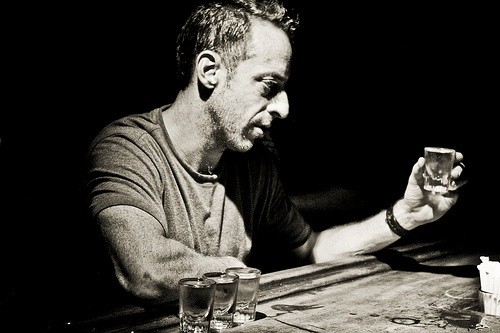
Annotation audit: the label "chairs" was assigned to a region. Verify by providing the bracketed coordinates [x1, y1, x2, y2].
[290, 181, 356, 231]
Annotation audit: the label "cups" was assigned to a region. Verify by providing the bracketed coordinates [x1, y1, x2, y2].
[202, 273, 239, 329]
[226, 267, 261, 322]
[478, 288, 500, 317]
[423, 147, 454, 192]
[178, 278, 216, 333]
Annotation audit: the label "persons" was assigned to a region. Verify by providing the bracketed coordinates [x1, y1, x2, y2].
[70, 0, 467, 327]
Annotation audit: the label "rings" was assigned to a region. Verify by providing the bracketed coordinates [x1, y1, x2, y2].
[459, 162, 465, 170]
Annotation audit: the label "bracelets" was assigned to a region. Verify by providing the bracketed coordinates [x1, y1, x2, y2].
[386, 205, 412, 238]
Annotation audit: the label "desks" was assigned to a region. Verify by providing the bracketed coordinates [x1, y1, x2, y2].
[47, 241, 500, 333]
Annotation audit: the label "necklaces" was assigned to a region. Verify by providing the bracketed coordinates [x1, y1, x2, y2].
[185, 145, 228, 174]
